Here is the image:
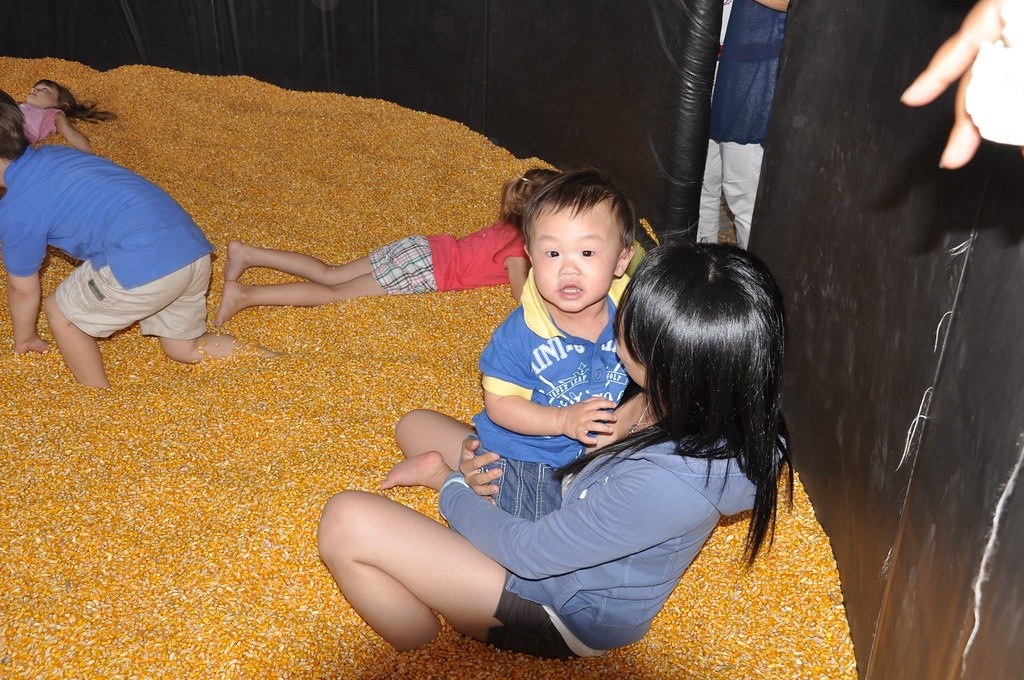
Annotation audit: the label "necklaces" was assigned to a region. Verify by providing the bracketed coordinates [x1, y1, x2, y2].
[628, 398, 659, 432]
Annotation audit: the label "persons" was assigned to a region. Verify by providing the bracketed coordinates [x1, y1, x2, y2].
[318, 239, 796, 653]
[901, 0, 1024, 171]
[691, 1, 790, 247]
[16, 79, 121, 154]
[377, 169, 638, 522]
[0, 88, 281, 389]
[217, 168, 560, 328]
